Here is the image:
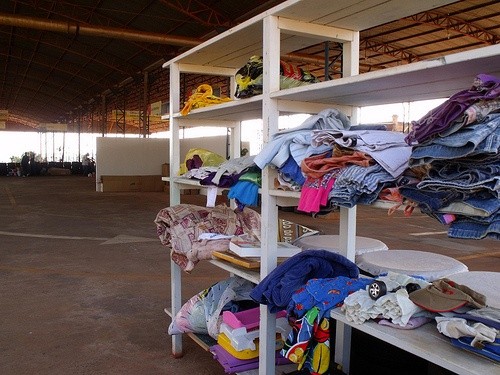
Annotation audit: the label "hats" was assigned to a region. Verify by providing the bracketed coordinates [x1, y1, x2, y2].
[409, 277, 488, 312]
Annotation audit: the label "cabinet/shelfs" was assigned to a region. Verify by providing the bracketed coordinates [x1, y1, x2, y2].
[161, 1, 500, 374]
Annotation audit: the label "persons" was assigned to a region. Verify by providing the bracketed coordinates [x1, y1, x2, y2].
[82, 153, 89, 177]
[21, 152, 29, 176]
